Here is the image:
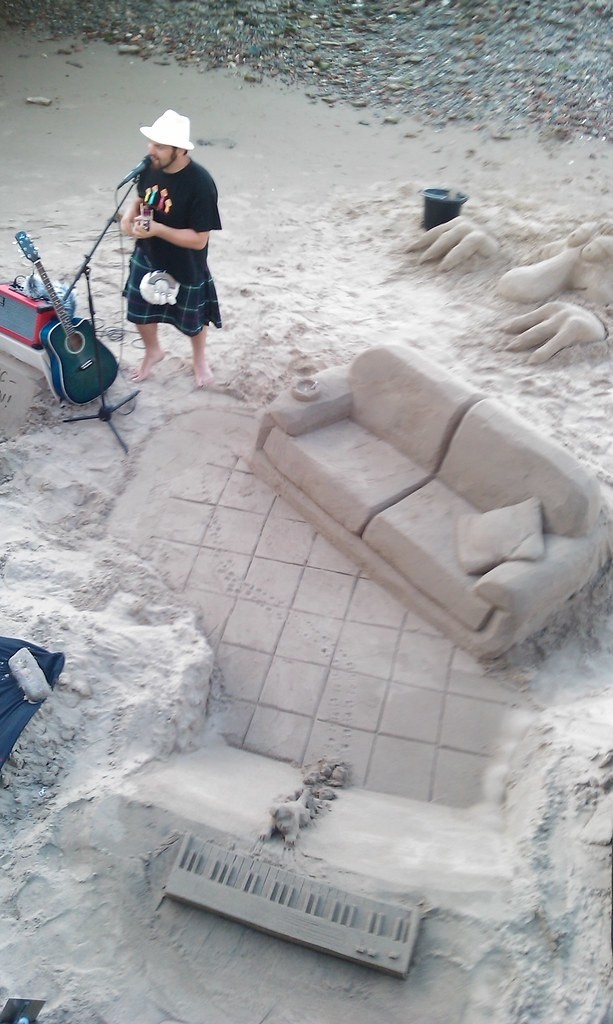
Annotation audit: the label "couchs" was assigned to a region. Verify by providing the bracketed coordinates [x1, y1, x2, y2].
[246, 341, 613, 663]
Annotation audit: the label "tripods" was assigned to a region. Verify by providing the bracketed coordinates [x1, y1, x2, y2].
[62, 175, 142, 454]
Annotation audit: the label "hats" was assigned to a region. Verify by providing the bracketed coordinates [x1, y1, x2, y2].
[139, 109, 195, 150]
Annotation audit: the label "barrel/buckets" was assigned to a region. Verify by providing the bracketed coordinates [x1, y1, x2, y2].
[420, 188, 468, 230]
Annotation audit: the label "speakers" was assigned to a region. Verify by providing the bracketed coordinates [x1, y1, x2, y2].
[0, 284, 57, 350]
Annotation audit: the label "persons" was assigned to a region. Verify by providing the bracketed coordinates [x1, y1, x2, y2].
[120, 109, 222, 386]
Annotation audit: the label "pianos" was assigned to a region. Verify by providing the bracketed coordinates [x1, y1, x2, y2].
[163, 828, 421, 980]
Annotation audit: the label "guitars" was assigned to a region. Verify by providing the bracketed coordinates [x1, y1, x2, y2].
[132, 202, 154, 234]
[16, 229, 119, 404]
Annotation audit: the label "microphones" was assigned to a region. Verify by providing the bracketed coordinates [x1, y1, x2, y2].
[119, 154, 153, 189]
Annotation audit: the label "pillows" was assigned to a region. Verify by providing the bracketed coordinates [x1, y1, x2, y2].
[458, 497, 547, 573]
